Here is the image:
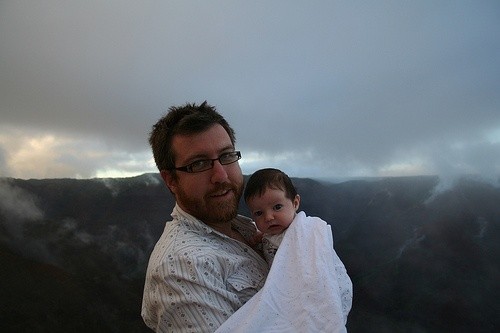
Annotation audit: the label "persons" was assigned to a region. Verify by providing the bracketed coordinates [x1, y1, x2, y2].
[243, 169, 352, 333]
[140, 99, 269, 333]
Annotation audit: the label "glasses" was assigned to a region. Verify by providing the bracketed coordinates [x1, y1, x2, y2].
[172, 151, 242, 173]
